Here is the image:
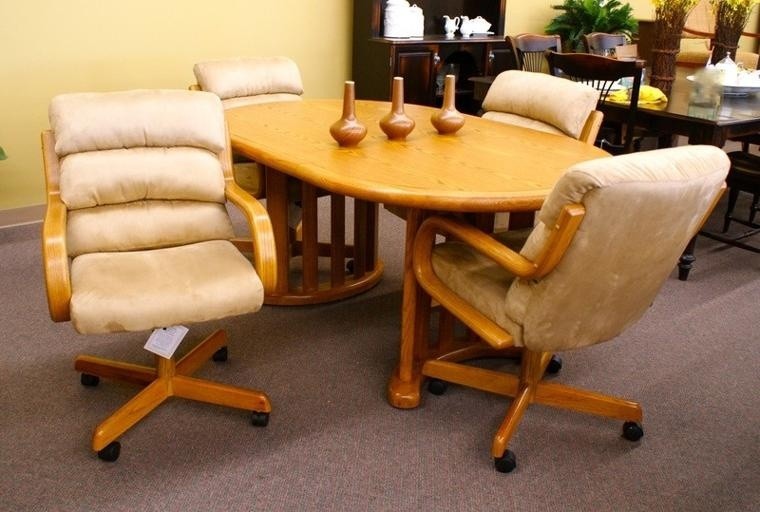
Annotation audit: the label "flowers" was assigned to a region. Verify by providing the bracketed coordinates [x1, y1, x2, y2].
[651, 0, 700, 31]
[711, 1, 760, 29]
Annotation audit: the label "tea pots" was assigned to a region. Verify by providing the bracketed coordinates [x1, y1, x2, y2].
[461, 16, 477, 38]
[443, 15, 460, 40]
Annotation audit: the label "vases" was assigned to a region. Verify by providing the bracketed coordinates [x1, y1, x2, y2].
[650, 29, 682, 93]
[711, 24, 742, 66]
[381, 76, 415, 141]
[431, 72, 464, 135]
[330, 81, 367, 147]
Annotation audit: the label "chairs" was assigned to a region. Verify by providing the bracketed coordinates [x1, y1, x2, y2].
[481, 69, 604, 146]
[504, 34, 562, 75]
[411, 144, 731, 472]
[543, 47, 648, 155]
[579, 33, 628, 61]
[40, 89, 277, 461]
[189, 55, 303, 200]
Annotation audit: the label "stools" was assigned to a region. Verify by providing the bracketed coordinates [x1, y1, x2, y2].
[697, 136, 760, 257]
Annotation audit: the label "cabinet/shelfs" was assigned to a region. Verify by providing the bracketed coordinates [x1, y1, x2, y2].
[352, 0, 507, 112]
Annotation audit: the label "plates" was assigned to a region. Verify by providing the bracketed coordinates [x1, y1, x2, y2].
[685, 75, 760, 97]
